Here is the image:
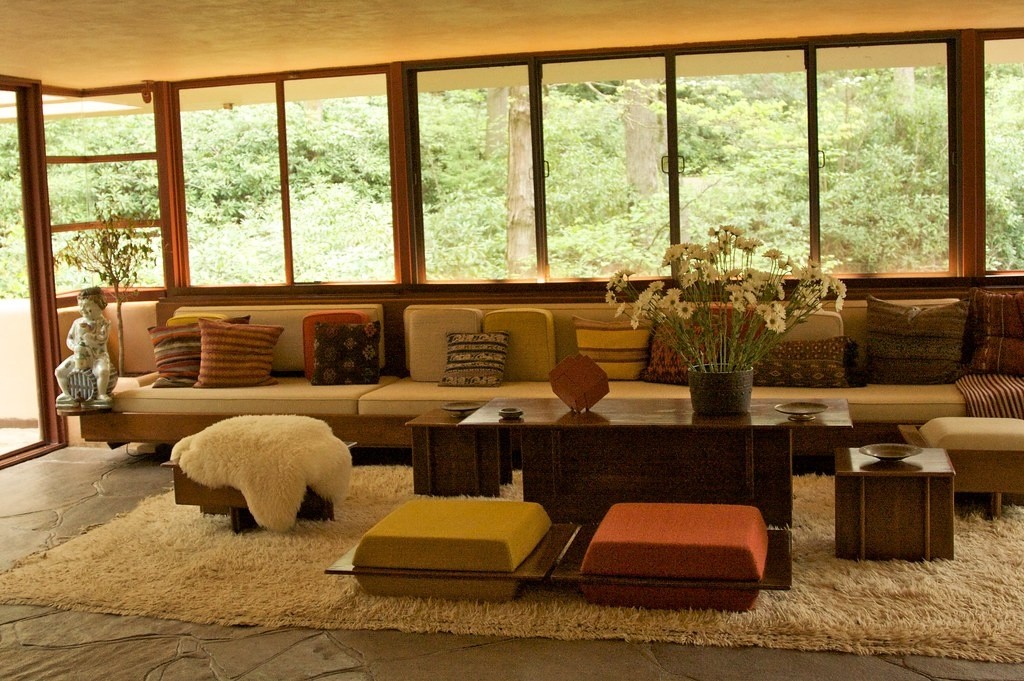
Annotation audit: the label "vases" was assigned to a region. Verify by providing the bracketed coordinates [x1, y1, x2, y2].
[688, 362, 755, 414]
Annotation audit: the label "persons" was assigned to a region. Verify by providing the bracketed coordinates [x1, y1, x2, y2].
[56, 285, 112, 401]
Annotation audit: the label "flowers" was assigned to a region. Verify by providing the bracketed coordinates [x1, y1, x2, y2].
[604, 222, 847, 372]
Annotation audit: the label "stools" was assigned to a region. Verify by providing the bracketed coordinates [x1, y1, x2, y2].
[324, 498, 582, 602]
[549, 502, 794, 609]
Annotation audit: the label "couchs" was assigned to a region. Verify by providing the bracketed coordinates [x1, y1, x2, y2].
[116, 296, 1024, 470]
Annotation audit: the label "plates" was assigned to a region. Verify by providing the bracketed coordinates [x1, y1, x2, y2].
[440, 402, 480, 417]
[859, 444, 923, 462]
[774, 402, 828, 421]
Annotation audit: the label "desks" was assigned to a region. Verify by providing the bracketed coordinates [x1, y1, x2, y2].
[161, 461, 328, 536]
[831, 446, 958, 563]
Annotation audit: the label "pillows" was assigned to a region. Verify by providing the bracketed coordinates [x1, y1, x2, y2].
[966, 284, 1024, 377]
[165, 315, 228, 324]
[310, 319, 383, 385]
[436, 329, 512, 389]
[754, 308, 846, 390]
[410, 308, 483, 382]
[192, 317, 284, 389]
[850, 292, 972, 387]
[485, 307, 557, 383]
[568, 313, 655, 382]
[145, 323, 202, 389]
[302, 309, 370, 381]
[641, 308, 763, 386]
[222, 314, 251, 325]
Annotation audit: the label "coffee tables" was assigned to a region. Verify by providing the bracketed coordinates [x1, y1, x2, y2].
[404, 397, 801, 593]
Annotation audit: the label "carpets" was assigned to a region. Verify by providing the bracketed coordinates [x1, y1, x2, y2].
[0, 465, 1024, 666]
